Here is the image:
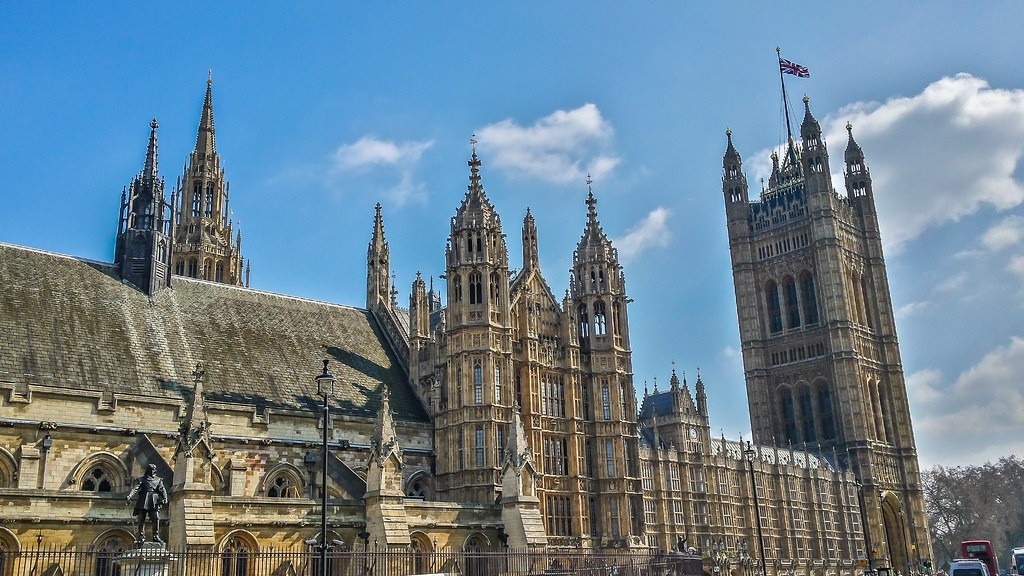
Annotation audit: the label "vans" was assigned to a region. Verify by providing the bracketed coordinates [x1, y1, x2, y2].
[950, 559, 986, 576]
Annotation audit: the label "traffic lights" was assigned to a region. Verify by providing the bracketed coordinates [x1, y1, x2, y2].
[928, 563, 931, 571]
[923, 562, 926, 569]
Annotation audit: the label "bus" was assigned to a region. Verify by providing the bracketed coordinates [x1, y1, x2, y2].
[961, 540, 999, 576]
[1011, 549, 1024, 576]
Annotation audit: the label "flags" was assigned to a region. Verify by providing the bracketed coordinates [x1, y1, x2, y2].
[780, 58, 810, 78]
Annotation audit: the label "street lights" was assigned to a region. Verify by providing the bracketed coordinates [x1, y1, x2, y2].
[924, 528, 931, 563]
[744, 441, 767, 576]
[358, 532, 370, 576]
[314, 359, 337, 576]
[853, 480, 871, 571]
[878, 494, 893, 567]
[912, 521, 921, 565]
[898, 510, 912, 576]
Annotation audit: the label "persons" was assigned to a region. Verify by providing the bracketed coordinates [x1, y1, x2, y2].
[678, 537, 688, 552]
[127, 464, 167, 543]
